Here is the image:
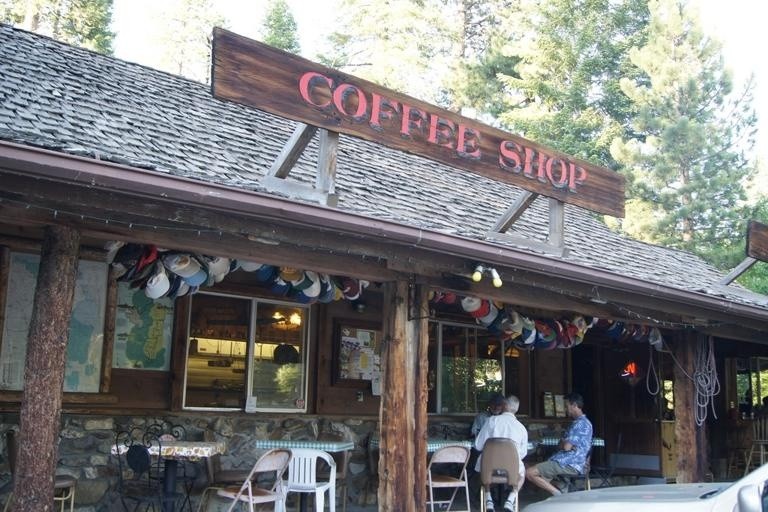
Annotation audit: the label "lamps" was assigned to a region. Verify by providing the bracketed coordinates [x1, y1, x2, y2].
[471, 265, 485, 283]
[490, 269, 503, 288]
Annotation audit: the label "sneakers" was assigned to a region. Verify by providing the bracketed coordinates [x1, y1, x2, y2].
[504, 500, 514, 512]
[486, 500, 496, 512]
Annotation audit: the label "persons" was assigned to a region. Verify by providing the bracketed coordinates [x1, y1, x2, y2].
[526, 392, 593, 495]
[471, 394, 504, 436]
[474, 394, 528, 512]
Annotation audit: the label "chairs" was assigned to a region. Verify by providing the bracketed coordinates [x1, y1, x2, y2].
[425, 445, 471, 512]
[3, 429, 78, 511]
[479, 437, 520, 512]
[216, 448, 293, 512]
[116, 427, 185, 512]
[274, 447, 336, 512]
[361, 431, 380, 507]
[557, 456, 591, 491]
[139, 413, 198, 512]
[308, 433, 347, 512]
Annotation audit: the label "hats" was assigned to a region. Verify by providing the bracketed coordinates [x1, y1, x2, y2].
[241, 259, 369, 303]
[105, 240, 240, 300]
[428, 287, 662, 351]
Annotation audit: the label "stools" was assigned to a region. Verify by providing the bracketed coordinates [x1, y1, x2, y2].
[726, 448, 754, 481]
[751, 417, 768, 442]
[593, 466, 616, 487]
[744, 441, 768, 478]
